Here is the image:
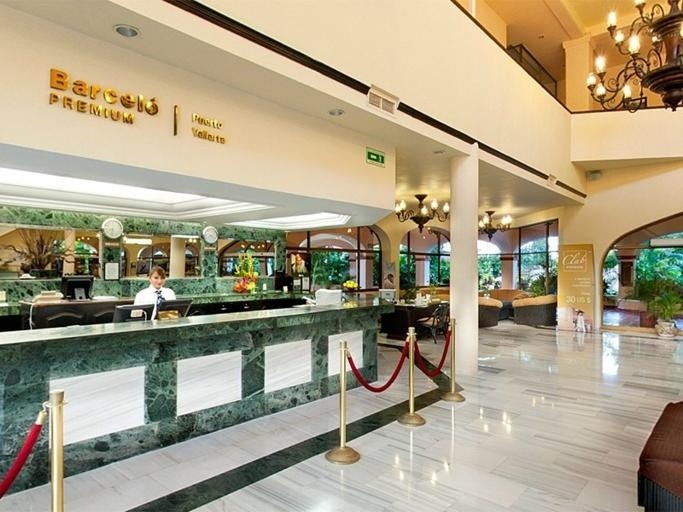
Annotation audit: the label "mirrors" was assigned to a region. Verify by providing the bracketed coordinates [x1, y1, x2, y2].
[118, 232, 203, 278]
[215, 237, 276, 278]
[0, 222, 99, 278]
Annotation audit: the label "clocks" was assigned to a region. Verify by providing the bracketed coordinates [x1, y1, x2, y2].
[100, 217, 123, 241]
[202, 225, 218, 245]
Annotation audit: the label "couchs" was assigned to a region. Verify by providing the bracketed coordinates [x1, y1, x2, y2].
[383, 282, 556, 343]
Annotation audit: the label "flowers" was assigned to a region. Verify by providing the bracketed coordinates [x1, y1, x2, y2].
[233, 253, 245, 277]
[232, 254, 257, 294]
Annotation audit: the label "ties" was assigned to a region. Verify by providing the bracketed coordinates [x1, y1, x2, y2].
[154, 290, 165, 304]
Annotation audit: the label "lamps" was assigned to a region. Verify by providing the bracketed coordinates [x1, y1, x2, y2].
[389, 193, 451, 235]
[579, 1, 682, 115]
[475, 208, 514, 242]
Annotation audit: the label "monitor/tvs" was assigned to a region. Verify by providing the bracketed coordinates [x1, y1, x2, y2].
[113, 304, 154, 324]
[155, 300, 193, 318]
[60, 275, 94, 302]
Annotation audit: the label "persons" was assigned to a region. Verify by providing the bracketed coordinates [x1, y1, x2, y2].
[291, 253, 308, 273]
[576, 311, 586, 333]
[383, 274, 396, 289]
[133, 266, 176, 306]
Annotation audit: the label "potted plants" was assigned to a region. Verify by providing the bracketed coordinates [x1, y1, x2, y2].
[645, 296, 683, 338]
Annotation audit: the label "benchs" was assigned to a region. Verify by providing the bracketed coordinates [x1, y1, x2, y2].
[632, 397, 682, 511]
